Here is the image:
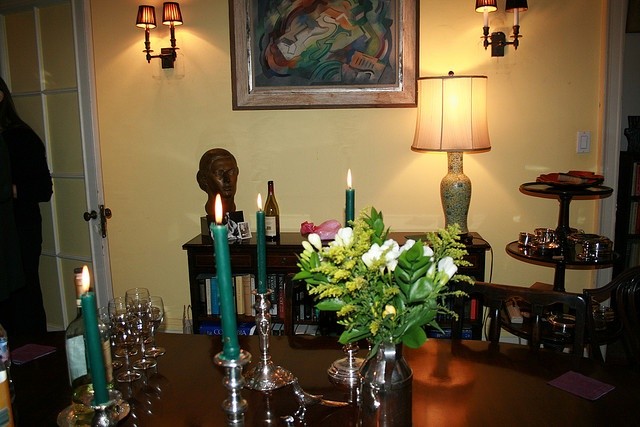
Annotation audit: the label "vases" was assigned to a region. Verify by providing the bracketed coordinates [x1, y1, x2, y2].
[359, 337, 414, 426]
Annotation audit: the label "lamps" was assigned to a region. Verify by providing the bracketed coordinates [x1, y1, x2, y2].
[411, 71, 491, 236]
[475, 0, 528, 57]
[136, 2, 183, 69]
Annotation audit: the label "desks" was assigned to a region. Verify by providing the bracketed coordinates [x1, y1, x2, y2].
[8, 330, 640, 426]
[183, 231, 491, 334]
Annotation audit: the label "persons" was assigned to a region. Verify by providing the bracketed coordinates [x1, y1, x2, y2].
[197, 146, 240, 217]
[1, 79, 54, 350]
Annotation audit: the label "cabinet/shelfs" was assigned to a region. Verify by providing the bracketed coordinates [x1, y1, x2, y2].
[619, 115, 640, 271]
[499, 171, 623, 348]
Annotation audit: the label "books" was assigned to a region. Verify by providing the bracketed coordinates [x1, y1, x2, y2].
[626, 160, 639, 269]
[204, 271, 322, 322]
[253, 323, 318, 337]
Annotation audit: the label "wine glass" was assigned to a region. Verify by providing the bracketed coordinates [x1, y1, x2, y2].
[98, 288, 168, 384]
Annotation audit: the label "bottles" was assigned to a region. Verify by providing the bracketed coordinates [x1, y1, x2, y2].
[262, 181, 280, 240]
[65, 268, 109, 392]
[1, 325, 16, 403]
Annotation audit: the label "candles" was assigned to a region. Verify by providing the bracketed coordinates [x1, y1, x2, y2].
[81, 265, 110, 406]
[345, 169, 355, 230]
[210, 193, 241, 363]
[256, 193, 267, 293]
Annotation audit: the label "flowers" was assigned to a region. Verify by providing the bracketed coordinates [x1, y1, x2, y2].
[292, 206, 478, 363]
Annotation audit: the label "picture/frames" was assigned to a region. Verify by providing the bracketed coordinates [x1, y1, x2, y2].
[228, 0, 420, 110]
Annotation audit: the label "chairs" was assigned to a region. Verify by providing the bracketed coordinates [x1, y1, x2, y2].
[583, 265, 640, 374]
[450, 281, 586, 356]
